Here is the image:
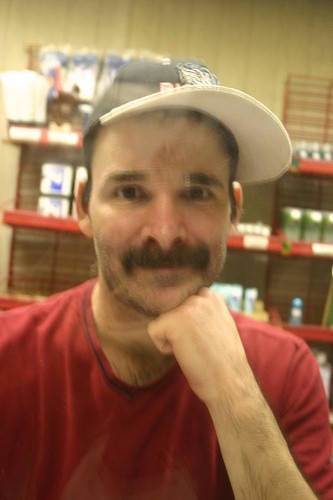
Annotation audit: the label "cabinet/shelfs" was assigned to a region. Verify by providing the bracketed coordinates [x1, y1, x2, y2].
[1, 125, 332, 428]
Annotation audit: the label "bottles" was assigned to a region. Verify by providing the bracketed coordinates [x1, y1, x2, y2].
[316, 352, 332, 412]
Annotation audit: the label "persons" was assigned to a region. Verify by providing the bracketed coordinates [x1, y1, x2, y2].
[1, 54, 332, 500]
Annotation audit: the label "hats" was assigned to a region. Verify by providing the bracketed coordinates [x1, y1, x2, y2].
[81, 55, 294, 187]
